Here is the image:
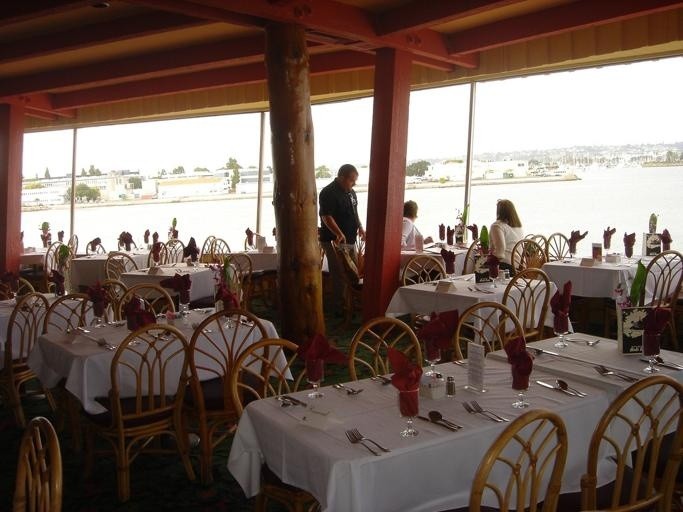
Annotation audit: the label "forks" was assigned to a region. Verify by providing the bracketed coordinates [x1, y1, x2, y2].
[461, 400, 503, 424]
[593, 364, 639, 382]
[351, 427, 392, 452]
[344, 428, 378, 456]
[470, 398, 508, 422]
[565, 336, 600, 347]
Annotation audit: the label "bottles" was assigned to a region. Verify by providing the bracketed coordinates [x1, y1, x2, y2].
[445, 377, 456, 397]
[480, 226, 489, 249]
[430, 373, 447, 399]
[497, 269, 510, 281]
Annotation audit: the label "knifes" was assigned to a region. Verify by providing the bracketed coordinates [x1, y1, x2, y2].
[286, 396, 306, 407]
[638, 357, 680, 371]
[418, 415, 455, 433]
[534, 378, 575, 398]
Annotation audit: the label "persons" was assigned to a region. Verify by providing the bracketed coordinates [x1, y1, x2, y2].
[487, 198, 526, 278]
[401, 200, 423, 251]
[319, 164, 366, 334]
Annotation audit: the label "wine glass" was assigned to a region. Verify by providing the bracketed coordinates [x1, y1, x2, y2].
[304, 359, 323, 398]
[511, 373, 530, 410]
[41, 231, 253, 271]
[178, 290, 190, 317]
[641, 332, 661, 373]
[398, 389, 420, 438]
[92, 303, 106, 329]
[222, 303, 235, 331]
[568, 238, 672, 265]
[436, 224, 456, 283]
[423, 344, 440, 376]
[553, 317, 568, 348]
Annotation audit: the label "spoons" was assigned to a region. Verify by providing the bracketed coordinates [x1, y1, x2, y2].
[654, 354, 681, 367]
[557, 379, 588, 395]
[430, 410, 462, 427]
[372, 374, 392, 386]
[333, 383, 363, 395]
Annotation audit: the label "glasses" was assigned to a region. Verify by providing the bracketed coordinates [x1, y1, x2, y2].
[348, 193, 356, 206]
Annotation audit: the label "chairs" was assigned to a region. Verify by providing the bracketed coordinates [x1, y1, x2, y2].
[2, 225, 276, 424]
[82, 320, 191, 499]
[465, 407, 569, 511]
[453, 301, 528, 361]
[347, 315, 423, 382]
[317, 230, 683, 348]
[10, 413, 67, 511]
[230, 336, 313, 420]
[577, 373, 682, 511]
[180, 308, 270, 486]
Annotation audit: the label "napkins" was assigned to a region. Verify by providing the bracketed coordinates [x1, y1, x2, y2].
[636, 305, 673, 359]
[383, 346, 432, 419]
[499, 334, 540, 394]
